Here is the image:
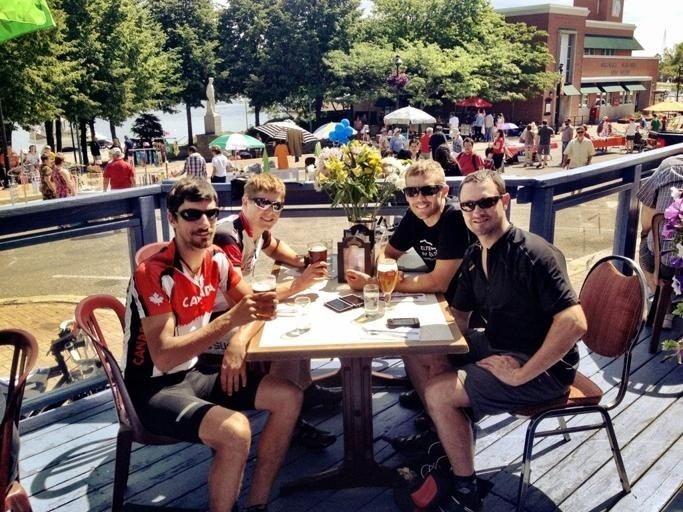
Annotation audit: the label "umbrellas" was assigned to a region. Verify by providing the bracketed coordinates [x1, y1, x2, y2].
[644, 99, 683, 118]
[455, 95, 494, 111]
[0, 1, 56, 47]
[383, 105, 437, 138]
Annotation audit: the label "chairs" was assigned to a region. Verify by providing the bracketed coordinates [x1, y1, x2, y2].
[647, 212, 683, 355]
[77, 279, 238, 511]
[510, 252, 644, 512]
[0, 324, 50, 512]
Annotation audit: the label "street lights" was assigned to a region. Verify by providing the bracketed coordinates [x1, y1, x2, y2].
[553, 61, 563, 135]
[391, 54, 402, 135]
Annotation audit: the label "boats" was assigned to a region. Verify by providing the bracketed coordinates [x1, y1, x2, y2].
[20, 117, 124, 172]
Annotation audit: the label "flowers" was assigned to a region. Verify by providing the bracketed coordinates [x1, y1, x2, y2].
[661, 200, 682, 369]
[318, 133, 413, 228]
[386, 69, 408, 86]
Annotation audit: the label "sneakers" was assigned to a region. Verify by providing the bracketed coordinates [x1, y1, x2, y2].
[389, 433, 436, 453]
[429, 489, 483, 512]
[291, 420, 337, 448]
[398, 389, 424, 407]
[302, 381, 342, 408]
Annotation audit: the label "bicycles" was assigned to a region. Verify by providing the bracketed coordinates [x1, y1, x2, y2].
[0, 319, 116, 423]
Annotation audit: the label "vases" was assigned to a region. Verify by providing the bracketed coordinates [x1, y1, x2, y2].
[348, 218, 377, 274]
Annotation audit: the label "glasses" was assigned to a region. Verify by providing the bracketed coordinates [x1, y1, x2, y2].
[460, 197, 503, 209]
[248, 196, 283, 212]
[174, 208, 221, 220]
[404, 186, 443, 196]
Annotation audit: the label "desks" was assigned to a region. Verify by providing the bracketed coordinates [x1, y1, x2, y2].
[223, 134, 624, 188]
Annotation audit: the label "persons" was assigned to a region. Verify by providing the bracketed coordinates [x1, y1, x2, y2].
[211, 167, 345, 449]
[406, 167, 591, 512]
[636, 154, 683, 329]
[343, 153, 483, 413]
[119, 175, 312, 511]
[1, 113, 683, 229]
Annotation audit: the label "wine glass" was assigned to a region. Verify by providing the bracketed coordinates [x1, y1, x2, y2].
[374, 257, 399, 312]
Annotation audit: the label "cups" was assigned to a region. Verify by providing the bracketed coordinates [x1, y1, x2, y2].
[362, 283, 380, 313]
[293, 295, 311, 331]
[248, 273, 276, 321]
[304, 241, 329, 281]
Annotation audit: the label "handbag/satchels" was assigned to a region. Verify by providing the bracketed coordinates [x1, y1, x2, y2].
[394, 442, 451, 512]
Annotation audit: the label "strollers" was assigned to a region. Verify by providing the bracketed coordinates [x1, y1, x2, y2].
[632, 132, 652, 153]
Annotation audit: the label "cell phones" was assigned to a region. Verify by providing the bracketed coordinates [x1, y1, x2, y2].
[339, 294, 364, 308]
[325, 298, 353, 312]
[387, 317, 419, 328]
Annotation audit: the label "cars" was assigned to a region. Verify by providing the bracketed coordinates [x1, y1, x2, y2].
[245, 117, 309, 148]
